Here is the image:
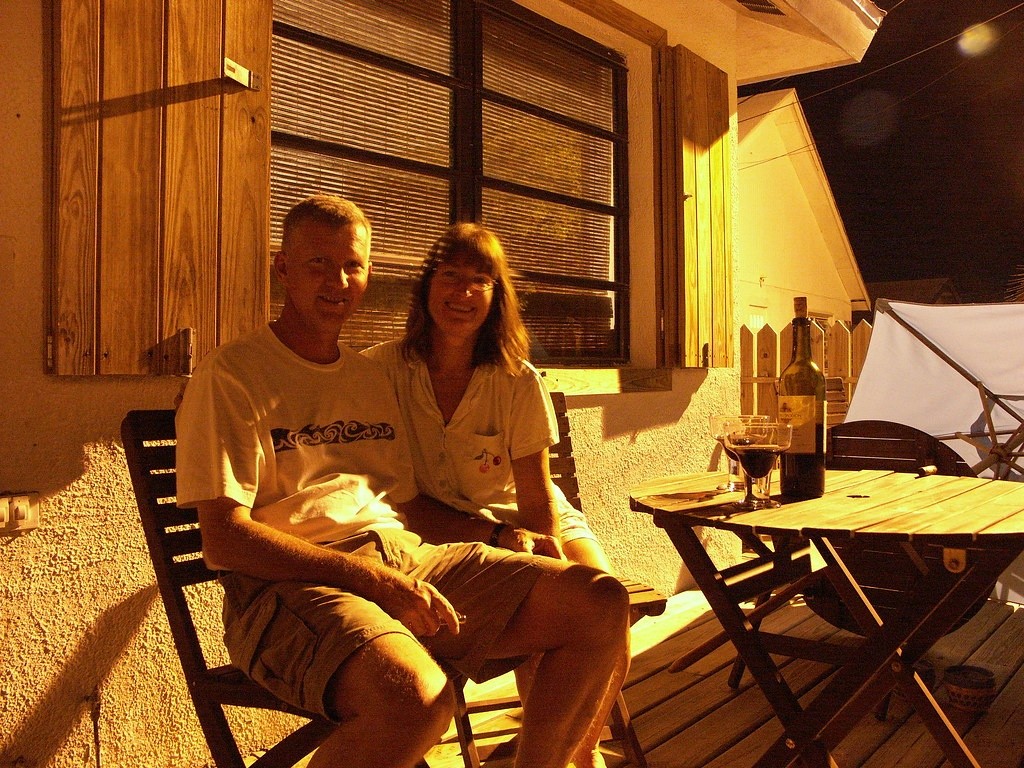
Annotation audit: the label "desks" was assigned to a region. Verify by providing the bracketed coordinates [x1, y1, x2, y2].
[628, 466, 1024, 768]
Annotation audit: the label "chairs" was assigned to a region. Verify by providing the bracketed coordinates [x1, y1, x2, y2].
[118, 391, 670, 768]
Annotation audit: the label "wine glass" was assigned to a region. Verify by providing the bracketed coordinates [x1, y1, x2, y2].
[724, 424, 792, 509]
[709, 414, 770, 488]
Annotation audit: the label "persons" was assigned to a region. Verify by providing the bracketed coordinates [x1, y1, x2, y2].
[360, 222, 619, 768]
[175, 195, 630, 768]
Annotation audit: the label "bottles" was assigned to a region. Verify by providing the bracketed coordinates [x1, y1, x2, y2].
[778, 297, 826, 499]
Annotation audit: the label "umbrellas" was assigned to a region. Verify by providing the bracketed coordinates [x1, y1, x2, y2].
[834, 298, 1024, 605]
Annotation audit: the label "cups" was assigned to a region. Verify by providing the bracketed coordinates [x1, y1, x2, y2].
[744, 470, 771, 500]
[727, 458, 743, 492]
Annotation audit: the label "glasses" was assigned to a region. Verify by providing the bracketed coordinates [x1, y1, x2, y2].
[433, 267, 498, 292]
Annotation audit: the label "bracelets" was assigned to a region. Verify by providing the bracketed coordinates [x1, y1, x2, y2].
[489, 519, 515, 547]
